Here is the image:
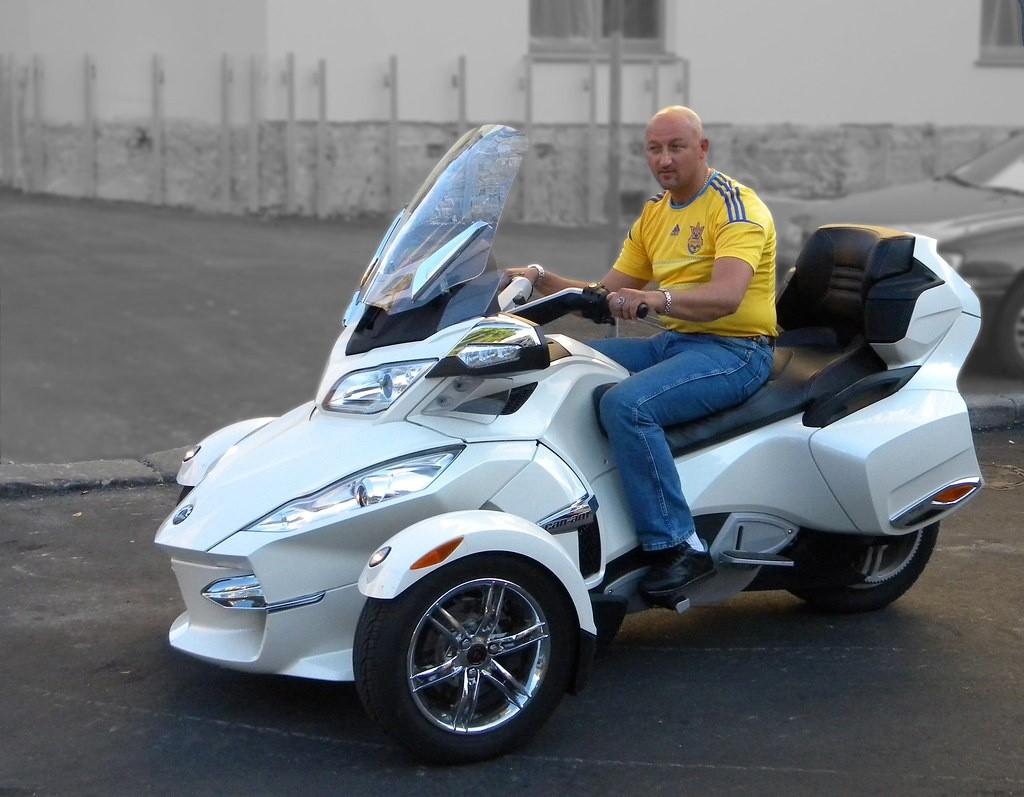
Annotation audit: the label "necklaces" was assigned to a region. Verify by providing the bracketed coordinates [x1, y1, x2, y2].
[703, 167, 711, 186]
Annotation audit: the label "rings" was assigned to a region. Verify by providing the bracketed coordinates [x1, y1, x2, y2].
[618, 296, 626, 306]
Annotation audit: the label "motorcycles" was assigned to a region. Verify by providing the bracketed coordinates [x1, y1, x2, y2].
[153, 123, 992, 765]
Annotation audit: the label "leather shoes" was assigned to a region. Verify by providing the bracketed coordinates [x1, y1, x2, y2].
[638, 538, 719, 596]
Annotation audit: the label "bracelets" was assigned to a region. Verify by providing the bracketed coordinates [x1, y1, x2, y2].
[527, 263, 545, 288]
[653, 289, 672, 315]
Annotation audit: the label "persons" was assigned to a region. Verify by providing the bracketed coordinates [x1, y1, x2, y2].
[493, 105, 779, 599]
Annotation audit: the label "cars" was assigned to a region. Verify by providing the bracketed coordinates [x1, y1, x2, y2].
[783, 129, 1024, 374]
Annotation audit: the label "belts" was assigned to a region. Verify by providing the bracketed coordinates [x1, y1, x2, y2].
[736, 336, 773, 349]
